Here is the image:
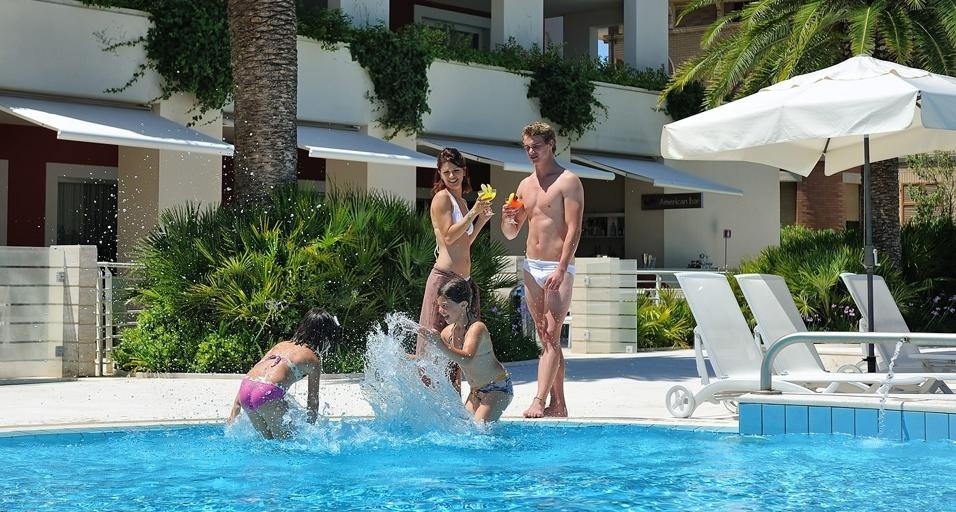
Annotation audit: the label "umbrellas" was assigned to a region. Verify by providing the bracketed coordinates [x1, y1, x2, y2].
[661, 54, 956, 372]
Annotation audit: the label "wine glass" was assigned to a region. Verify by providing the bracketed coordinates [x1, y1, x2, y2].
[505, 198, 519, 225]
[477, 189, 497, 217]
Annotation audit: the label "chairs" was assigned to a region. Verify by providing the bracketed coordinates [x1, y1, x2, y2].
[666, 273, 956, 418]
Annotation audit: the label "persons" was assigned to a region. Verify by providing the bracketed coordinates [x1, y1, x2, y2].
[416, 149, 493, 399]
[500, 122, 584, 417]
[406, 278, 513, 422]
[227, 308, 335, 440]
